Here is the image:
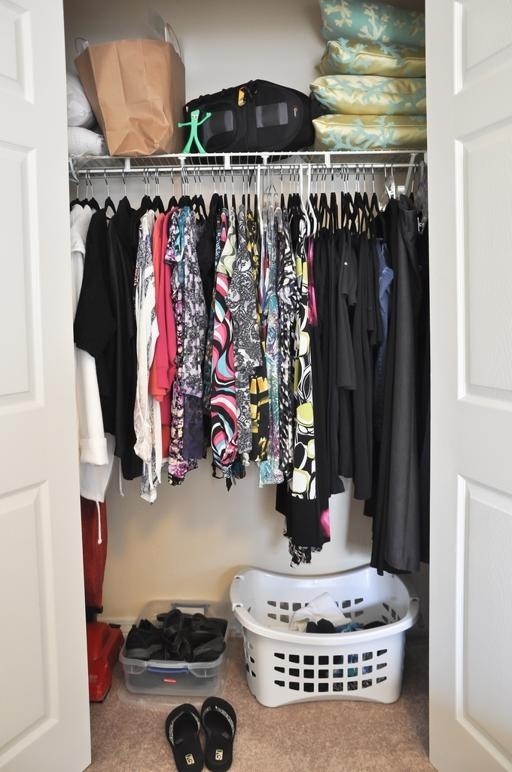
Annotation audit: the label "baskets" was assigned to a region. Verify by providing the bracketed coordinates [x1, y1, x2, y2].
[230, 563, 419, 707]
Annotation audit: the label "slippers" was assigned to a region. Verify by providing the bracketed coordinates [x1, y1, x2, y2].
[202, 697, 236, 772]
[166, 705, 203, 772]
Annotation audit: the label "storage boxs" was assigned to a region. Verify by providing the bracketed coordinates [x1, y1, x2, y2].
[118, 600, 232, 709]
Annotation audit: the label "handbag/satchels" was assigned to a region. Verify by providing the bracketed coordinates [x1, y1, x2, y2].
[184, 80, 314, 164]
[74, 24, 185, 156]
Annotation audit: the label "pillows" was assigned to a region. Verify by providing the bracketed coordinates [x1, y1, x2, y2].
[298, 1, 427, 164]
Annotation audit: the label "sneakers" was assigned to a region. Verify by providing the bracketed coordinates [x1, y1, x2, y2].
[127, 608, 227, 660]
